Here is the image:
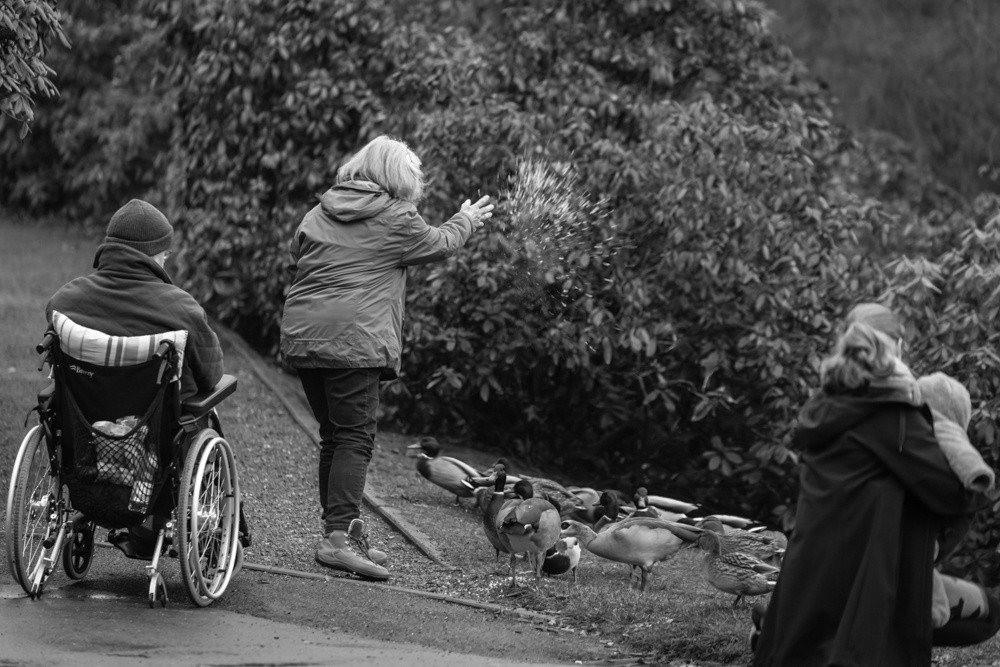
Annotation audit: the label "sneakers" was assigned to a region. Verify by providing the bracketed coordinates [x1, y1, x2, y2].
[314, 519, 389, 580]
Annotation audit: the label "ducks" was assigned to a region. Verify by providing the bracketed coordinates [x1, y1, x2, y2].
[406, 436, 787, 611]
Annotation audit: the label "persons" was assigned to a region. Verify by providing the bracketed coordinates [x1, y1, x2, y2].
[746, 304, 996, 667]
[45, 197, 254, 549]
[280, 133, 494, 577]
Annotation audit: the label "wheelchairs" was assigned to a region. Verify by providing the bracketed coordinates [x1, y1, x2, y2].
[7, 307, 248, 613]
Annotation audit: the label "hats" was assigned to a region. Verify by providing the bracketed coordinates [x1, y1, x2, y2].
[104, 198, 174, 256]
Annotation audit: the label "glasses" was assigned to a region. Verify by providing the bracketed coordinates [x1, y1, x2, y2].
[164, 248, 173, 260]
